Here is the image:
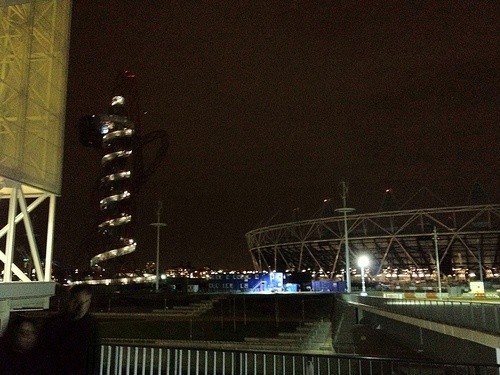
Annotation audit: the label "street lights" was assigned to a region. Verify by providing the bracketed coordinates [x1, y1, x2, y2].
[149, 199, 168, 291]
[432, 225, 443, 293]
[333, 179, 356, 293]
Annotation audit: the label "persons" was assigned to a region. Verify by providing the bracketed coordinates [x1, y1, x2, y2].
[0, 314, 35, 375]
[35, 283, 98, 374]
